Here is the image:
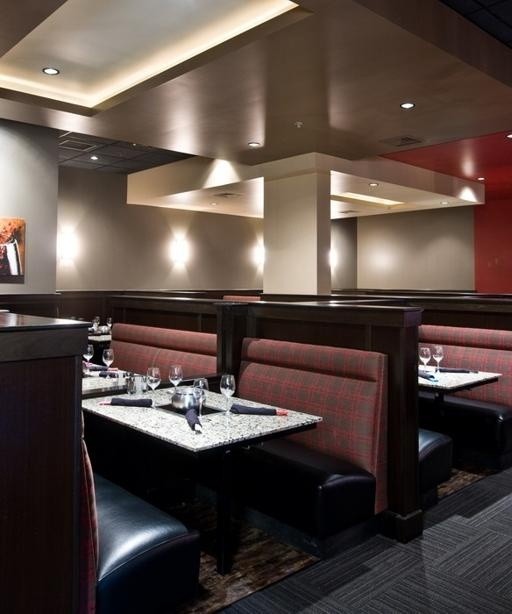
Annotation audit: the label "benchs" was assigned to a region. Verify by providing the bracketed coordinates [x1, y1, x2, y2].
[56, 319, 389, 611]
[416, 322, 512, 507]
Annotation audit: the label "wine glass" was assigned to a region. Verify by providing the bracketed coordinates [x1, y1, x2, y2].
[431, 345, 445, 377]
[418, 347, 430, 379]
[220, 374, 236, 415]
[146, 367, 161, 407]
[102, 349, 115, 382]
[83, 344, 95, 375]
[193, 377, 210, 422]
[168, 364, 184, 400]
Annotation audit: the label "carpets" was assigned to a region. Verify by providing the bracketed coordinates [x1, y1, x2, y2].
[197, 467, 510, 614]
